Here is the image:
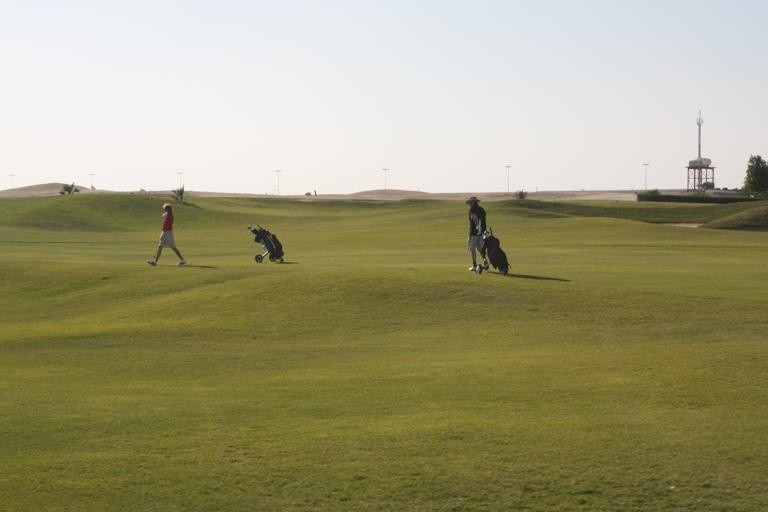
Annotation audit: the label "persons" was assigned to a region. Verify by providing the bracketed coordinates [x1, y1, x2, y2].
[145, 203, 186, 266]
[465, 195, 491, 271]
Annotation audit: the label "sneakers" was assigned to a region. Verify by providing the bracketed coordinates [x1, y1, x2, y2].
[176, 260, 186, 265]
[468, 259, 489, 271]
[146, 260, 157, 266]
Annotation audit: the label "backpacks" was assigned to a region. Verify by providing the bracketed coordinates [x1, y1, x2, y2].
[254, 228, 284, 261]
[483, 236, 508, 275]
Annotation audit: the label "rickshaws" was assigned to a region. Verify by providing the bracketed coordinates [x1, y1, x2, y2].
[248, 225, 284, 263]
[475, 225, 509, 276]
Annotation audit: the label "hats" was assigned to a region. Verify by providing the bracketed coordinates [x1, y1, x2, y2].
[466, 197, 480, 204]
